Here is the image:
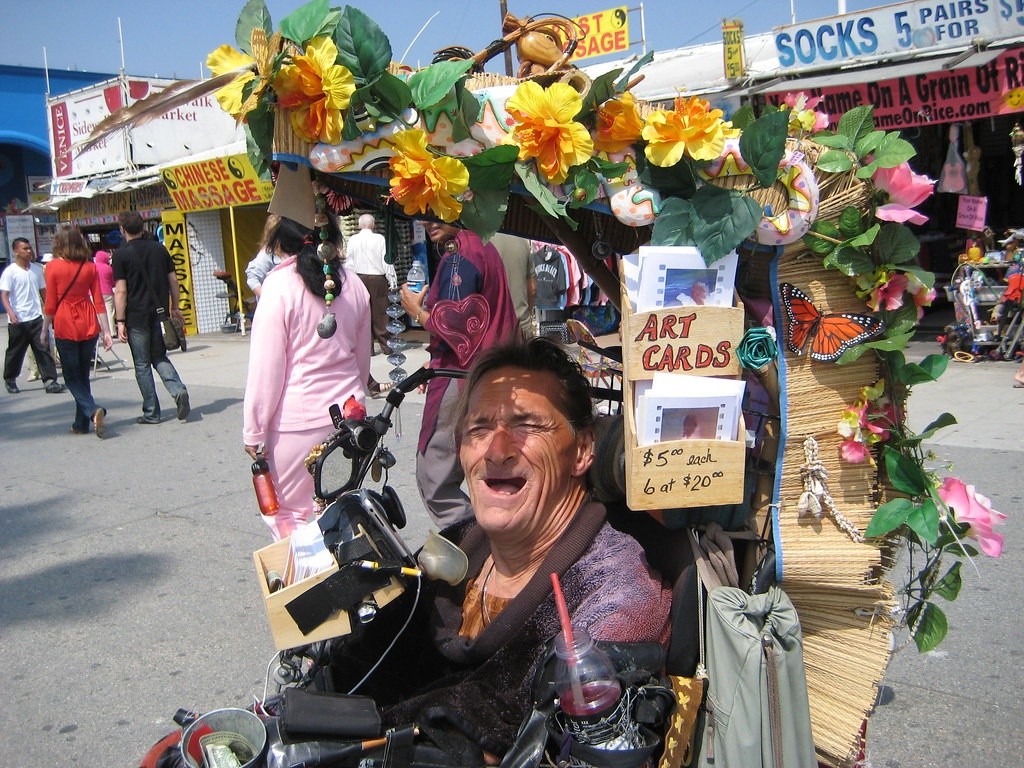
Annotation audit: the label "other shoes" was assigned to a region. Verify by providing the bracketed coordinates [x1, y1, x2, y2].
[28, 374, 38, 381]
[70, 427, 84, 434]
[94, 407, 106, 438]
[136, 416, 151, 424]
[176, 390, 190, 420]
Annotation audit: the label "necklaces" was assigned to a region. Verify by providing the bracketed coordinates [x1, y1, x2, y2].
[480, 561, 495, 628]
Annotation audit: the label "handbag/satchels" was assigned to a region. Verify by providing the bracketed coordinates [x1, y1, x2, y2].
[159, 316, 187, 352]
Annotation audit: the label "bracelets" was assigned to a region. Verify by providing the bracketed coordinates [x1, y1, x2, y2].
[117, 320, 126, 322]
[415, 310, 425, 324]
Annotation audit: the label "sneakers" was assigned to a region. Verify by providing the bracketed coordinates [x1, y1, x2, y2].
[5, 383, 18, 393]
[47, 382, 65, 393]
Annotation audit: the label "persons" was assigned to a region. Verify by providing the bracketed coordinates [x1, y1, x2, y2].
[489, 233, 538, 337]
[401, 221, 523, 530]
[0, 238, 64, 394]
[244, 205, 370, 541]
[112, 211, 190, 424]
[246, 214, 283, 303]
[41, 226, 114, 438]
[305, 339, 674, 768]
[95, 251, 118, 338]
[346, 214, 398, 357]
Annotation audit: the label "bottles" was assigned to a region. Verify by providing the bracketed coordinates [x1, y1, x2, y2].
[251, 452, 279, 515]
[554, 627, 645, 750]
[407, 261, 424, 294]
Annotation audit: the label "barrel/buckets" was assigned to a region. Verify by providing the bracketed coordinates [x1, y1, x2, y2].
[180, 708, 266, 768]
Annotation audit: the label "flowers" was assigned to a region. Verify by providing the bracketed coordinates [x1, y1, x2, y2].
[204, 0, 1006, 650]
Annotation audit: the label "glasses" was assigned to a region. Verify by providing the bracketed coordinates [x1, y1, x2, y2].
[342, 212, 413, 286]
